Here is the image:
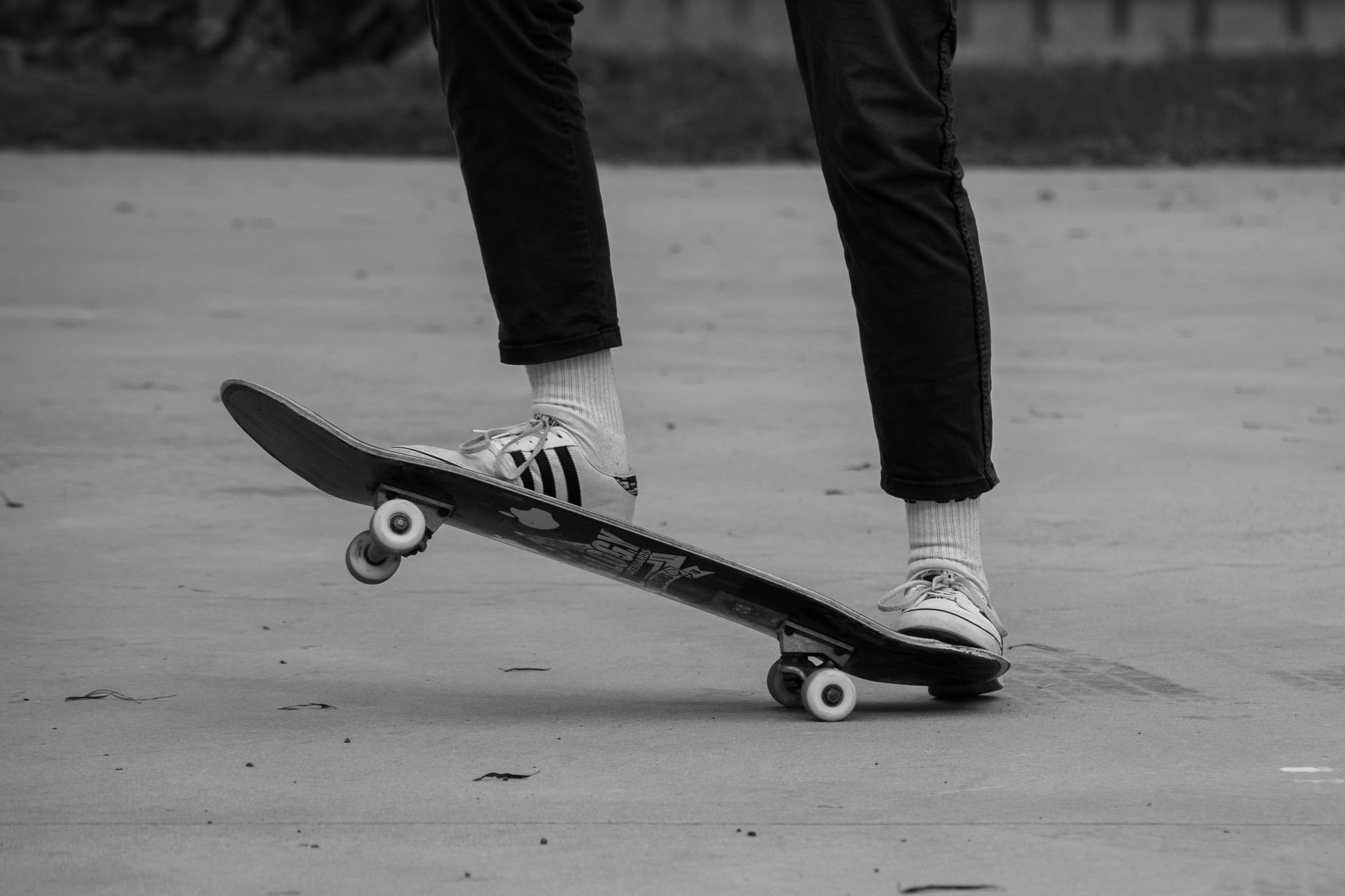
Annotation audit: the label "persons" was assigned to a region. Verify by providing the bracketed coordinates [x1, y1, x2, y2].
[384, 0, 1007, 658]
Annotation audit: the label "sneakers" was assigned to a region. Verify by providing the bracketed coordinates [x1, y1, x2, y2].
[893, 570, 1005, 660]
[388, 415, 638, 526]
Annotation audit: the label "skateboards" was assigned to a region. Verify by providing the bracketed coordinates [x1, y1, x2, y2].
[218, 372, 1012, 725]
